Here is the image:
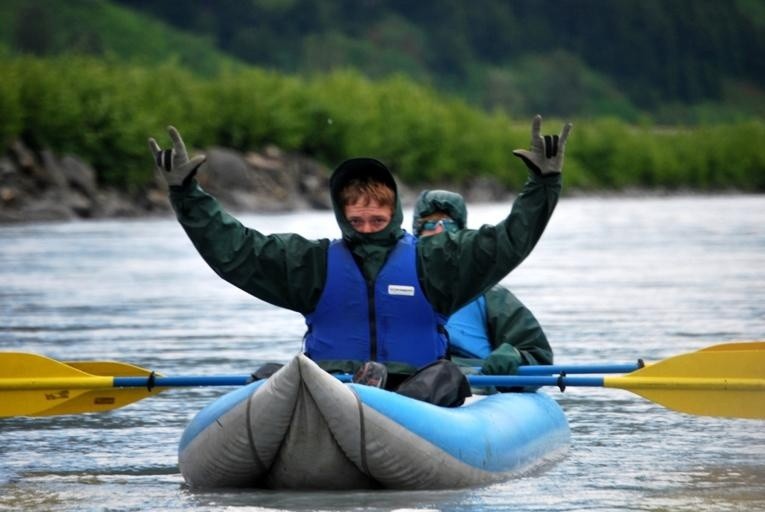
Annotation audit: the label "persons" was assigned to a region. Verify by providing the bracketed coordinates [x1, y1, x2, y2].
[148, 114, 574, 408]
[412, 188, 554, 395]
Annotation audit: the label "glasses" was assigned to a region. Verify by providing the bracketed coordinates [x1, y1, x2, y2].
[422, 218, 459, 230]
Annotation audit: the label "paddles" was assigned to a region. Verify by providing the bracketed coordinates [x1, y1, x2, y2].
[0, 341, 765, 419]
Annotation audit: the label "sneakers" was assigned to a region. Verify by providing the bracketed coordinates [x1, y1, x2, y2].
[353, 360, 388, 389]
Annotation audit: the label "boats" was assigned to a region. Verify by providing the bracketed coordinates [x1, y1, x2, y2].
[179, 354, 571, 492]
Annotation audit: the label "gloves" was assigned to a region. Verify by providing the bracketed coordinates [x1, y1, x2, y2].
[512, 114, 573, 183]
[147, 124, 208, 191]
[480, 342, 522, 375]
